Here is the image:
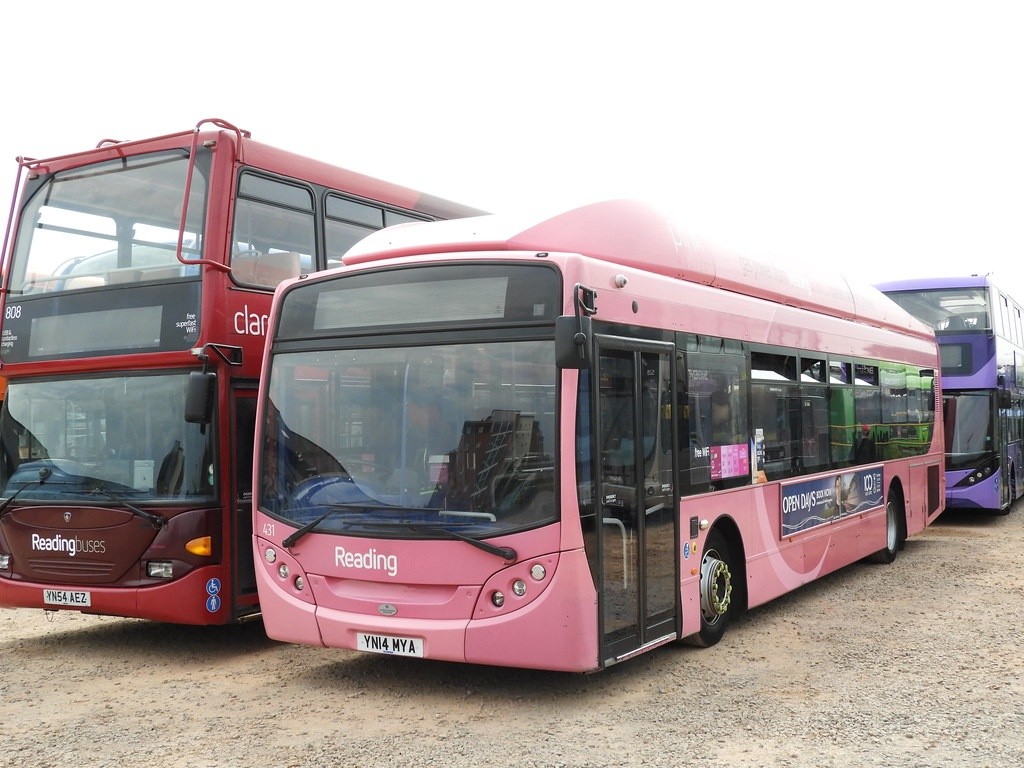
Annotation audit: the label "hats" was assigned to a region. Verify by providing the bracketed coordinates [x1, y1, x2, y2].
[862, 424, 869, 434]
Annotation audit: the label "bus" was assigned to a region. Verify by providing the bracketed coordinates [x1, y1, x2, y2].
[0, 117, 556, 628]
[250, 198, 948, 675]
[878, 274, 1024, 517]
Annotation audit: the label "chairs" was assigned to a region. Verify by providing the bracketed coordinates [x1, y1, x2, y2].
[105, 248, 302, 289]
[946, 311, 990, 331]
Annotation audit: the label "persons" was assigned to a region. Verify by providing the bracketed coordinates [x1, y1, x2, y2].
[833, 476, 848, 517]
[710, 391, 769, 443]
[849, 423, 878, 461]
